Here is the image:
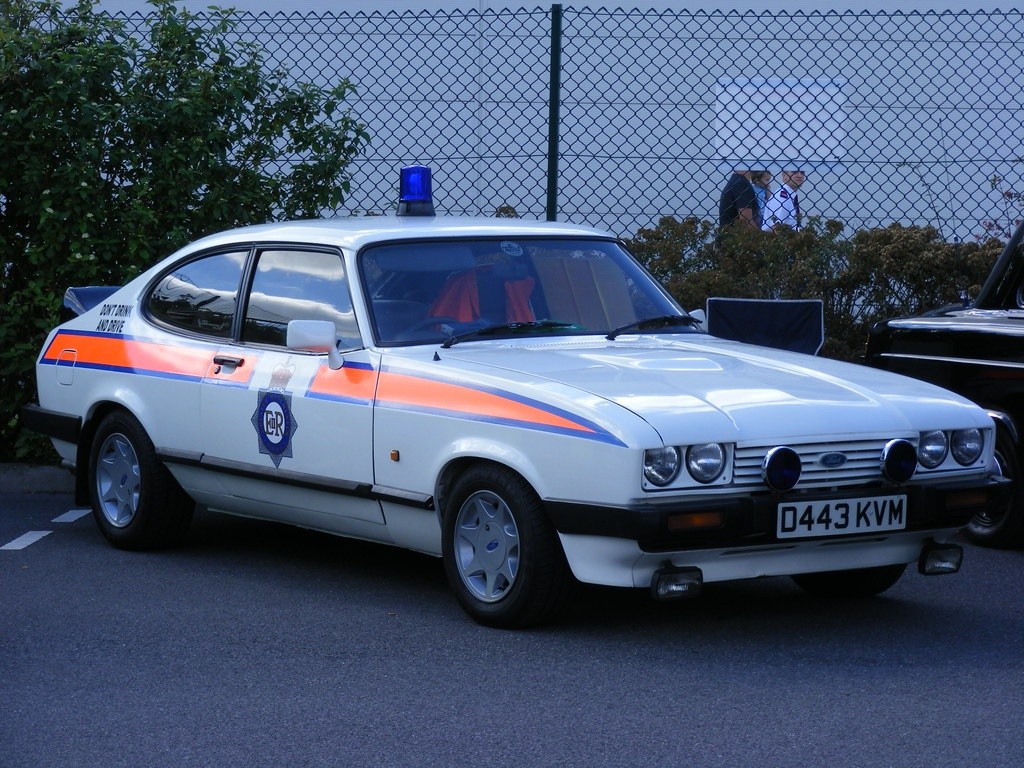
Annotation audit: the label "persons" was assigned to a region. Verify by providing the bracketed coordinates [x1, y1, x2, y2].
[716, 170, 809, 233]
[427, 241, 536, 334]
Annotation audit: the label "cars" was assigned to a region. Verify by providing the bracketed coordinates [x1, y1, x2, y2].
[861, 218, 1024, 548]
[17, 163, 1014, 632]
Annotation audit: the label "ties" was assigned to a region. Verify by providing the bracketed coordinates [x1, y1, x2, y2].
[793, 194, 801, 228]
